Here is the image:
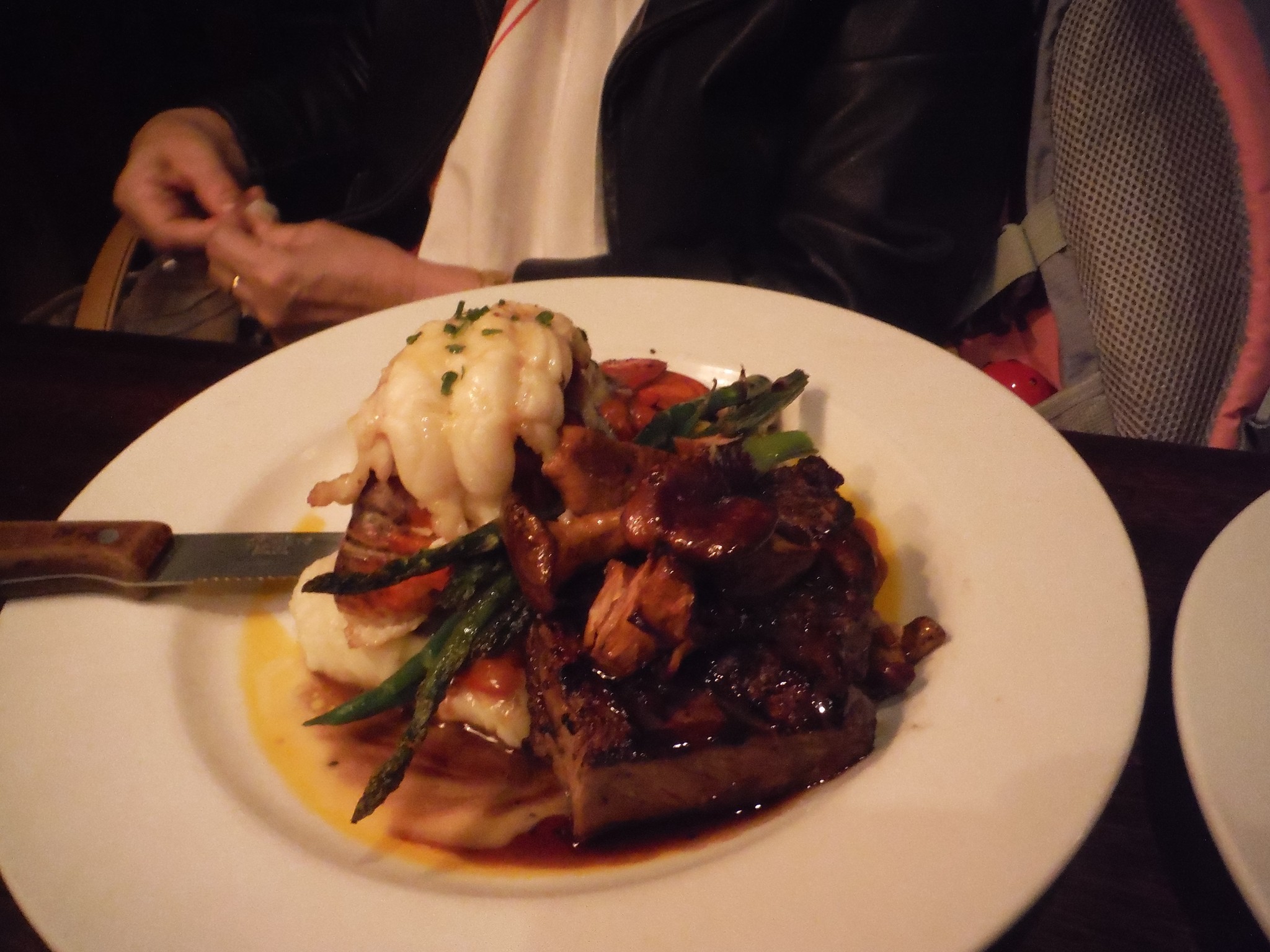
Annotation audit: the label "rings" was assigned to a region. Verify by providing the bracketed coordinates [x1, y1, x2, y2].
[227, 275, 240, 298]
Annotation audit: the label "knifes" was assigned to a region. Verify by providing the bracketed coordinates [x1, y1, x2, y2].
[1, 520, 345, 596]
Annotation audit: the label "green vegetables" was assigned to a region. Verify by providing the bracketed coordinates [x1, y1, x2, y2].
[405, 300, 557, 396]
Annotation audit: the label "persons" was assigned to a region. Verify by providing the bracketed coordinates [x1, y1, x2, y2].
[95, 0, 1045, 385]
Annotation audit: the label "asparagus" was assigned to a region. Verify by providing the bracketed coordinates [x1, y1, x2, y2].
[301, 369, 819, 827]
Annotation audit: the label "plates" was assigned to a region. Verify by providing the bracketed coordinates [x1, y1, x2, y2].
[1168, 492, 1270, 940]
[0, 275, 1151, 950]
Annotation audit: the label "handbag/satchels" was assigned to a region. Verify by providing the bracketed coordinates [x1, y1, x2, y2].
[23, 218, 241, 344]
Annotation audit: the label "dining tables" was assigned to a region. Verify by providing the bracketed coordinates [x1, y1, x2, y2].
[0, 317, 1270, 951]
